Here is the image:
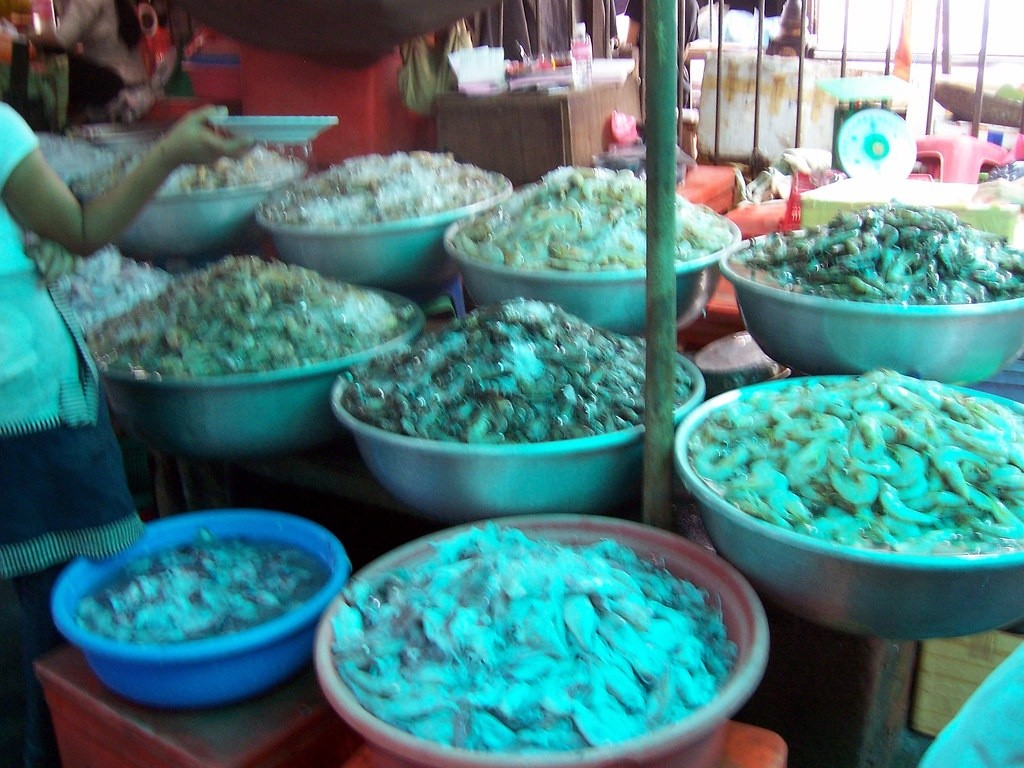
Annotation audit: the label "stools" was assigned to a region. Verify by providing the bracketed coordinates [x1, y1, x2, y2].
[908, 136, 1012, 184]
[33, 648, 788, 768]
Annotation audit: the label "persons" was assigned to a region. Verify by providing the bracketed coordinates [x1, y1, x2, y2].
[29, 0, 148, 119]
[765, 0, 816, 59]
[624, 0, 700, 108]
[0, 102, 256, 768]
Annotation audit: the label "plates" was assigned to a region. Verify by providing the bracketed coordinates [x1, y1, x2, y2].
[206, 115, 339, 141]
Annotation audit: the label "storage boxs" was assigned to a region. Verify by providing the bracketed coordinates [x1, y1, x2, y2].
[696, 48, 838, 168]
[433, 62, 642, 194]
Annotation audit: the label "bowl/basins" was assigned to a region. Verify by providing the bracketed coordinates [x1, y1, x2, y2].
[673, 375, 1024, 639]
[255, 178, 514, 289]
[99, 285, 427, 461]
[71, 153, 309, 257]
[48, 507, 351, 710]
[443, 214, 742, 336]
[717, 230, 1024, 384]
[328, 351, 707, 526]
[311, 512, 771, 768]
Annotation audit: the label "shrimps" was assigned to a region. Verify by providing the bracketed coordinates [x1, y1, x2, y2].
[744, 197, 1024, 303]
[330, 521, 737, 756]
[345, 297, 695, 443]
[688, 369, 1023, 550]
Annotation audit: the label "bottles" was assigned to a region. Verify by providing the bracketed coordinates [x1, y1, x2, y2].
[570, 22, 593, 92]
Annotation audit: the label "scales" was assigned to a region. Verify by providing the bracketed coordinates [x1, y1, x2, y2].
[816, 76, 917, 179]
